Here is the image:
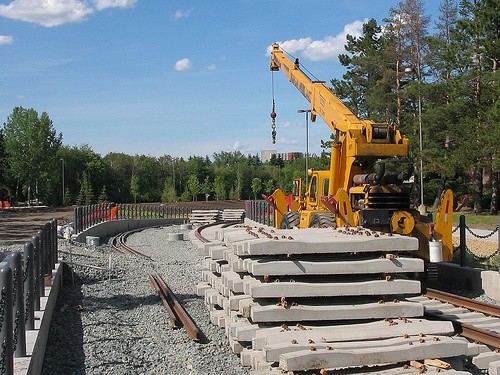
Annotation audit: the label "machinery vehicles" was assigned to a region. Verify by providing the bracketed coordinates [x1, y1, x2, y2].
[268, 42, 454, 293]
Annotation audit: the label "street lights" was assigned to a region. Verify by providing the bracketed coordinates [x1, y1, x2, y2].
[297, 109, 312, 193]
[61, 158, 65, 203]
